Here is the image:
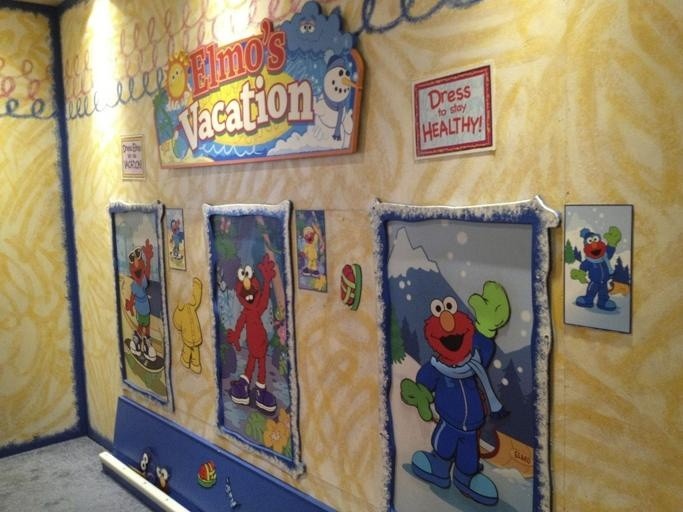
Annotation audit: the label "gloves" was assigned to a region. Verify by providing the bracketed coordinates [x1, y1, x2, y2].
[604, 224, 625, 247]
[400, 378, 434, 423]
[466, 279, 513, 338]
[570, 268, 589, 285]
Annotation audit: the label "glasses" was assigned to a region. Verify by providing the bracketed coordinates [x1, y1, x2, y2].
[127, 247, 143, 263]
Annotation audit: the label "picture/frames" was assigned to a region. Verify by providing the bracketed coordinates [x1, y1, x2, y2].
[563, 204, 635, 336]
[366, 197, 560, 512]
[105, 198, 187, 417]
[199, 200, 308, 482]
[410, 57, 496, 161]
[118, 133, 146, 181]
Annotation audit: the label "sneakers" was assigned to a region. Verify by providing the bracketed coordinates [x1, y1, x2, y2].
[576, 295, 618, 313]
[129, 339, 158, 361]
[411, 448, 503, 509]
[229, 374, 277, 413]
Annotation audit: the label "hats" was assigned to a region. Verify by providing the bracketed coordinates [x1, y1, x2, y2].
[579, 226, 601, 246]
